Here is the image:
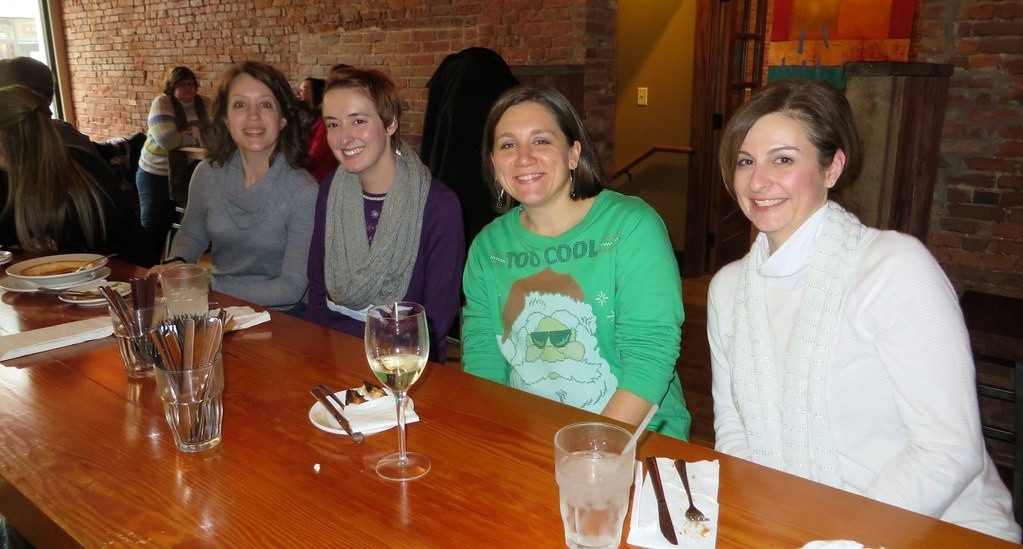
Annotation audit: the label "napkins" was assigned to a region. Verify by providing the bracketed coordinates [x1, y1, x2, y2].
[207, 305, 273, 330]
[343, 394, 421, 435]
[626, 456, 720, 549]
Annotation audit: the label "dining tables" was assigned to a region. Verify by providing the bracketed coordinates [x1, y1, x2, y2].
[0, 249, 1023, 549]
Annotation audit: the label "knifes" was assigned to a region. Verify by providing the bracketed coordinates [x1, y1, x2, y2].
[146, 306, 234, 445]
[309, 388, 364, 444]
[644, 456, 679, 545]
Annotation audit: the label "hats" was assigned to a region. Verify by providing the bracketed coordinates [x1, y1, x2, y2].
[1, 57, 53, 99]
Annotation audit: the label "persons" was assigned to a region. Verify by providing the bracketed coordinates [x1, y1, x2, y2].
[295, 69, 463, 364]
[0, 57, 141, 266]
[462, 84, 690, 443]
[707, 78, 1022, 544]
[293, 64, 359, 182]
[137, 66, 208, 271]
[143, 62, 319, 321]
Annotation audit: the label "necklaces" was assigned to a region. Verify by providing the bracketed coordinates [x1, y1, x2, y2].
[363, 149, 401, 201]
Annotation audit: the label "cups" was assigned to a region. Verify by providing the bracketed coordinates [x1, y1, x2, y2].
[553, 420, 636, 549]
[153, 352, 224, 453]
[108, 303, 155, 379]
[159, 263, 209, 319]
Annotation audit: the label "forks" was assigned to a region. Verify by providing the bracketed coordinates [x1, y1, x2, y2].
[673, 459, 711, 522]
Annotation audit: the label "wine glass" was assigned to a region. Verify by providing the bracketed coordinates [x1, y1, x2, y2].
[364, 301, 432, 482]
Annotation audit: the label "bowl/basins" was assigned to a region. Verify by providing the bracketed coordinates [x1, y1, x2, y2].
[5, 253, 108, 288]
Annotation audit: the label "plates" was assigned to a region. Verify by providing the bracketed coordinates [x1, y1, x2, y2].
[0, 266, 112, 293]
[58, 281, 132, 307]
[308, 386, 415, 437]
[0, 250, 13, 266]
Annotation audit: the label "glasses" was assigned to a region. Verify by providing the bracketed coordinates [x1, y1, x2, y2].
[178, 81, 195, 88]
